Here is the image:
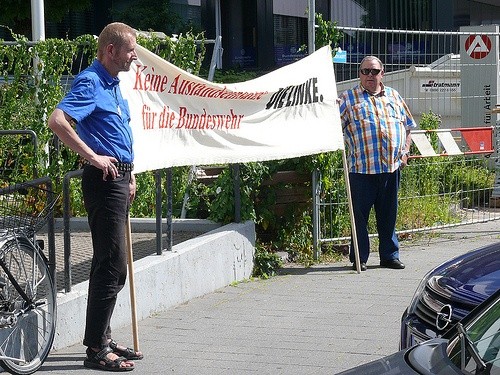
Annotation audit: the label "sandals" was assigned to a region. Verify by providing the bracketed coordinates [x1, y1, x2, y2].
[84, 346, 135, 371]
[109, 339, 143, 360]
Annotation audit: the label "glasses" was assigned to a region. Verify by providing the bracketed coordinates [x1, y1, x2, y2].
[361, 69, 380, 75]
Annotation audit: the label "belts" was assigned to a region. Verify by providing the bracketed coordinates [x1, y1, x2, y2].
[84, 158, 134, 171]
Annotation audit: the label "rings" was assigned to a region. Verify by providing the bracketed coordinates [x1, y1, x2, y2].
[110, 167, 112, 170]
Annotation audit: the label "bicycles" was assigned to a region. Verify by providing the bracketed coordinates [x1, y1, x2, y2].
[0, 174, 61, 375]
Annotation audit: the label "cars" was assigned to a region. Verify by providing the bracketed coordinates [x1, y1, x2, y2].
[398, 239, 500, 375]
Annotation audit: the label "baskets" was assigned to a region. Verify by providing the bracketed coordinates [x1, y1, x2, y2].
[0, 177, 59, 237]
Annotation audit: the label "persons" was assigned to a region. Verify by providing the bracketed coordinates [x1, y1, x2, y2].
[48, 22, 144, 372]
[338, 56, 417, 271]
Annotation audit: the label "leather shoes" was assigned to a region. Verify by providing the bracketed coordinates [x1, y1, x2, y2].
[380, 259, 405, 269]
[353, 262, 367, 271]
